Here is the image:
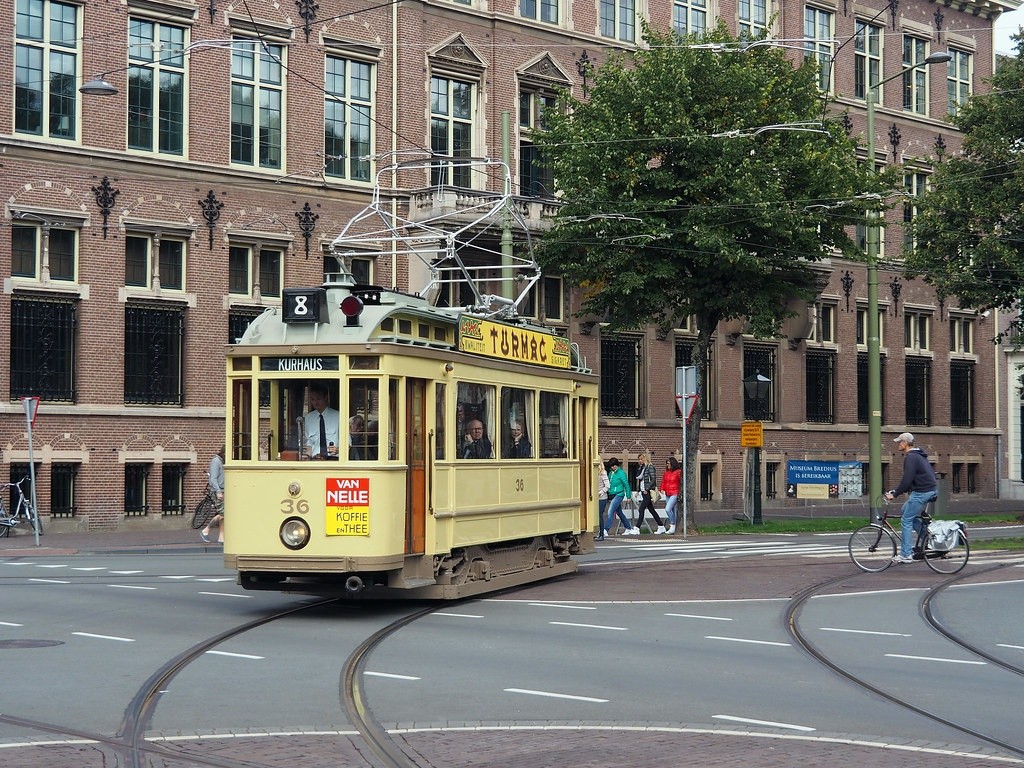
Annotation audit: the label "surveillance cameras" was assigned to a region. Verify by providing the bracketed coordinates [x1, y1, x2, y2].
[981, 310, 990, 319]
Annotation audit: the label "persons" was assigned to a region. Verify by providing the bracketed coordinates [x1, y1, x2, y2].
[660, 457, 682, 535]
[347, 413, 374, 460]
[502, 419, 531, 458]
[629, 454, 666, 535]
[200, 443, 226, 545]
[303, 381, 351, 460]
[462, 419, 492, 459]
[884, 433, 938, 564]
[594, 455, 611, 541]
[597, 457, 632, 536]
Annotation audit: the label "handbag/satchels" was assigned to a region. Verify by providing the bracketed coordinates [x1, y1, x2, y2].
[650, 487, 661, 503]
[926, 520, 967, 551]
[608, 490, 624, 500]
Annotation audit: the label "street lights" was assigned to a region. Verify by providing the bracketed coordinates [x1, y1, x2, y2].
[740, 367, 772, 524]
[867, 50, 952, 528]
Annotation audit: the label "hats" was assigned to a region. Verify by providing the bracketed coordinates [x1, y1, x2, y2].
[894, 433, 914, 442]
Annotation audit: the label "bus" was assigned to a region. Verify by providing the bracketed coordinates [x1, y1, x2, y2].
[222, 156, 601, 603]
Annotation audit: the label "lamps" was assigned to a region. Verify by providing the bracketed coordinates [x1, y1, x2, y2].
[78, 75, 119, 96]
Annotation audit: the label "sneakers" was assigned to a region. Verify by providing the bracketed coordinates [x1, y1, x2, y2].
[892, 554, 913, 564]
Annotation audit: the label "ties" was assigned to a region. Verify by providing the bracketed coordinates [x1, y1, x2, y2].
[319, 414, 327, 460]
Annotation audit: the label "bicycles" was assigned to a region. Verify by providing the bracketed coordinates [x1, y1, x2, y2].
[0, 474, 44, 538]
[848, 488, 969, 575]
[190, 471, 219, 529]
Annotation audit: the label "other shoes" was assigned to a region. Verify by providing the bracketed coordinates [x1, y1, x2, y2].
[593, 535, 604, 541]
[603, 529, 608, 536]
[200, 531, 211, 543]
[654, 525, 666, 535]
[665, 525, 676, 534]
[622, 529, 634, 536]
[218, 540, 224, 545]
[633, 526, 640, 535]
